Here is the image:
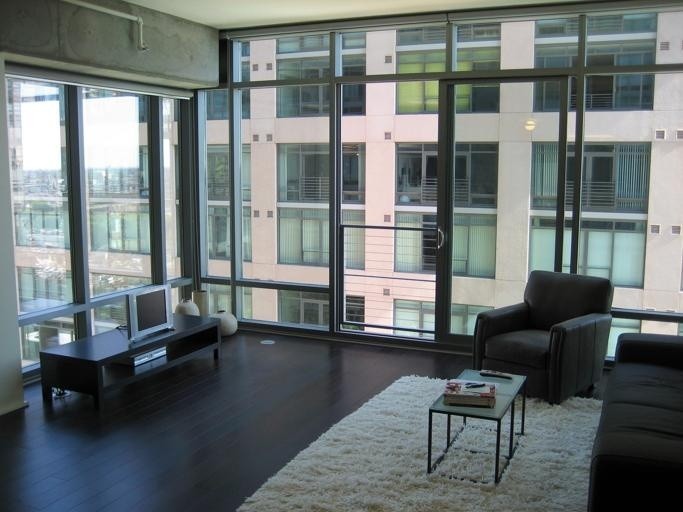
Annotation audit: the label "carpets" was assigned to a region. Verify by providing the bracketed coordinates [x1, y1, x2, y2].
[235, 374, 604, 512]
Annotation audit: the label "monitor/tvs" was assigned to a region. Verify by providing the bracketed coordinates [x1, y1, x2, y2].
[126, 283, 173, 340]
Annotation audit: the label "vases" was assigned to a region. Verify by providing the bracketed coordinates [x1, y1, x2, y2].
[176, 290, 238, 336]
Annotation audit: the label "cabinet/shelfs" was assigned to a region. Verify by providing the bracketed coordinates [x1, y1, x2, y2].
[39, 314, 222, 426]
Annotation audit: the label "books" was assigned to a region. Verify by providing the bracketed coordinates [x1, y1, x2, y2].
[442, 378, 497, 409]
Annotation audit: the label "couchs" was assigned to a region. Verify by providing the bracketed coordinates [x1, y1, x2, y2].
[587, 333, 683, 512]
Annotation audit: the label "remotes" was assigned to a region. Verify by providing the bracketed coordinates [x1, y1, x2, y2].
[480, 369, 513, 379]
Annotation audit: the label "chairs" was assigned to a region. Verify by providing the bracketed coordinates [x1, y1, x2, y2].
[474, 271, 614, 404]
